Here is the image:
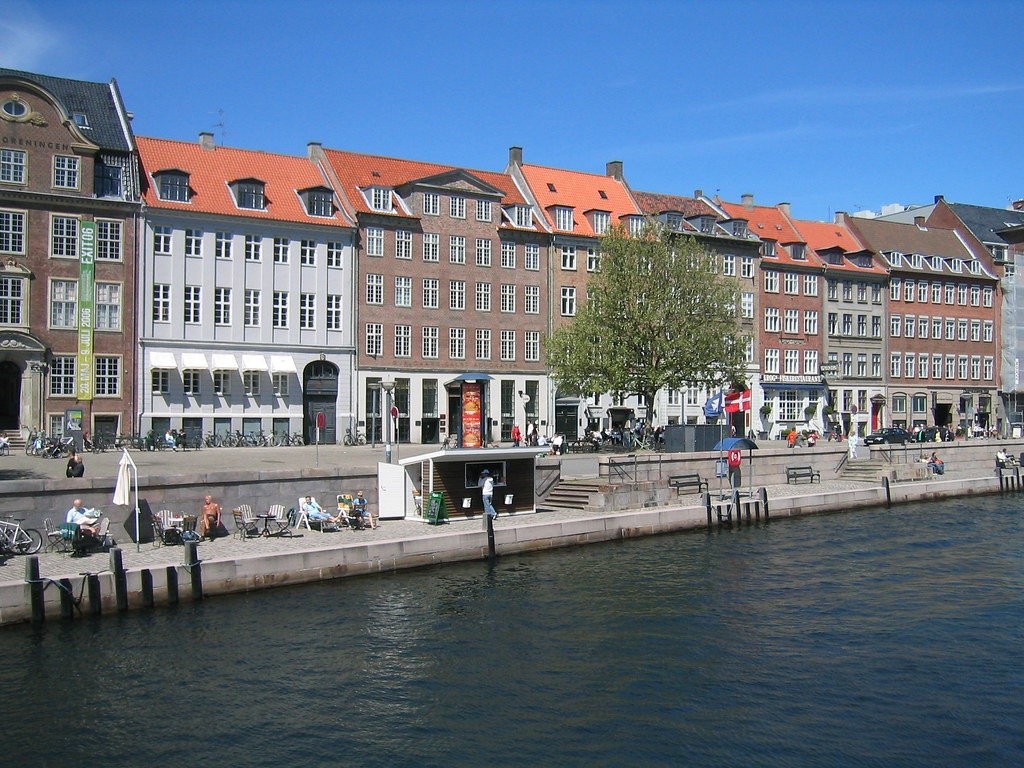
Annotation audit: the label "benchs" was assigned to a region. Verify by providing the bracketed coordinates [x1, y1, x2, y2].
[412, 490, 421, 517]
[785, 466, 821, 485]
[915, 459, 944, 473]
[667, 473, 708, 497]
[110, 436, 147, 450]
[995, 455, 1016, 469]
[158, 435, 202, 452]
[567, 439, 598, 453]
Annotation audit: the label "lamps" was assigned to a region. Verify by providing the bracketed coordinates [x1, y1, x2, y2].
[518, 390, 523, 397]
[30, 348, 53, 374]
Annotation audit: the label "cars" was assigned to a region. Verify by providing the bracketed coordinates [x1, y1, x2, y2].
[863, 427, 912, 446]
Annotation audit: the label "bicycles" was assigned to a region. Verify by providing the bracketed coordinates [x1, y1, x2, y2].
[343, 428, 367, 446]
[22, 424, 307, 460]
[439, 433, 457, 450]
[622, 427, 656, 453]
[0, 514, 43, 556]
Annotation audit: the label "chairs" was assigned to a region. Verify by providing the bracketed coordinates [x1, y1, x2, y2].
[149, 510, 198, 548]
[276, 508, 297, 539]
[296, 497, 341, 533]
[899, 431, 902, 434]
[337, 494, 379, 529]
[238, 504, 260, 534]
[232, 510, 255, 542]
[44, 506, 111, 558]
[267, 504, 285, 534]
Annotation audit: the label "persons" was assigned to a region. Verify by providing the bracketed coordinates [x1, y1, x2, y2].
[66, 499, 97, 556]
[31, 426, 62, 459]
[808, 430, 818, 447]
[352, 491, 377, 530]
[787, 428, 798, 448]
[65, 451, 85, 478]
[907, 423, 954, 443]
[849, 431, 858, 459]
[958, 424, 996, 440]
[303, 496, 339, 523]
[835, 422, 842, 441]
[478, 469, 497, 520]
[552, 432, 564, 455]
[0, 432, 9, 456]
[199, 496, 221, 542]
[919, 452, 944, 475]
[997, 448, 1016, 468]
[733, 453, 738, 462]
[889, 424, 903, 429]
[601, 422, 665, 446]
[511, 420, 550, 448]
[166, 430, 187, 452]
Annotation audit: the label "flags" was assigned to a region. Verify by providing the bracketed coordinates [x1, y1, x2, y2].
[703, 387, 723, 418]
[725, 389, 750, 413]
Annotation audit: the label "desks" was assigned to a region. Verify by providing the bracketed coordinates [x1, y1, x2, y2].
[256, 514, 277, 538]
[169, 518, 184, 536]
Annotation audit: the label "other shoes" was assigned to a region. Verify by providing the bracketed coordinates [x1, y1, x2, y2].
[493, 513, 497, 519]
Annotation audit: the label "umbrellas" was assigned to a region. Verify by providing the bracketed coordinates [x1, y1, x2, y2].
[112, 447, 140, 554]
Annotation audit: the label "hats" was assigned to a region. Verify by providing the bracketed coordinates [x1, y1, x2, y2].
[481, 470, 489, 473]
